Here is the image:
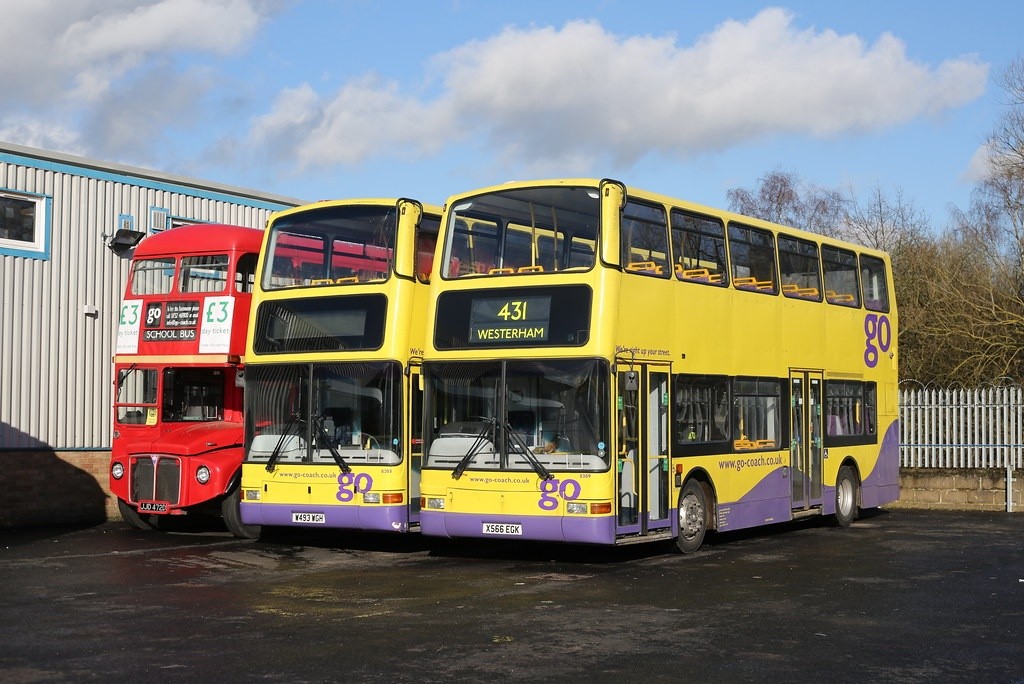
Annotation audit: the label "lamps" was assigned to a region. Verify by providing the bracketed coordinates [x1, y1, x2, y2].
[102, 229, 146, 255]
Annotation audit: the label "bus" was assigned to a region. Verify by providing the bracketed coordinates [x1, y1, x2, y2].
[420, 183, 900, 553]
[240, 196, 445, 533]
[109, 223, 267, 537]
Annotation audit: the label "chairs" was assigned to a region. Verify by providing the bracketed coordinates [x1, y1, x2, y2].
[305, 262, 883, 311]
